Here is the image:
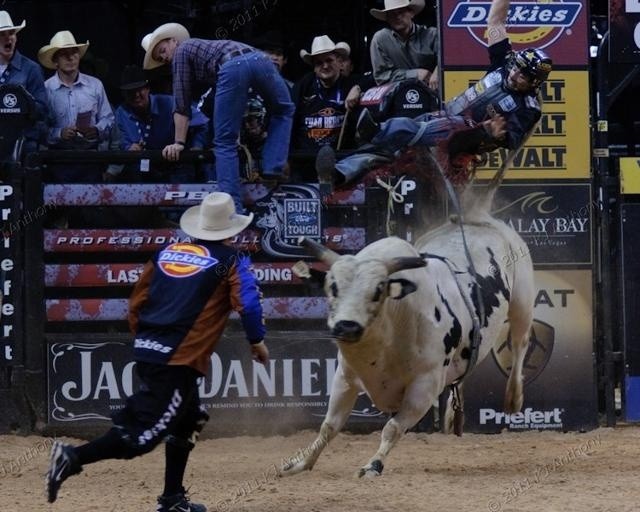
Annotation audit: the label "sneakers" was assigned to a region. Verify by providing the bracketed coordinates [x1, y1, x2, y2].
[46, 441, 84, 502]
[156, 488, 207, 512]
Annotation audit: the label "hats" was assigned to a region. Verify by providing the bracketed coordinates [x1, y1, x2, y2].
[140, 23, 192, 71]
[118, 64, 151, 90]
[181, 191, 255, 243]
[300, 35, 351, 64]
[368, 0, 426, 22]
[0, 10, 27, 34]
[36, 30, 91, 71]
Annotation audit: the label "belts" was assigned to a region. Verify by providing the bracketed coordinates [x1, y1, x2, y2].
[215, 47, 254, 65]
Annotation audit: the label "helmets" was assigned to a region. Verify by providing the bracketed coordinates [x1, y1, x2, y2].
[502, 46, 552, 95]
[243, 99, 264, 124]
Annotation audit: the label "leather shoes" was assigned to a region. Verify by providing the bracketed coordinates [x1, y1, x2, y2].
[354, 107, 378, 147]
[314, 144, 337, 196]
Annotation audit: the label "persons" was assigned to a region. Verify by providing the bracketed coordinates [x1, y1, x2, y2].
[332, 42, 353, 74]
[365, 1, 441, 95]
[317, 1, 552, 183]
[301, 35, 364, 179]
[44, 192, 270, 512]
[0, 10, 298, 215]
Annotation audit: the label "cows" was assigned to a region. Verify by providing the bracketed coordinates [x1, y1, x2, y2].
[275, 86, 544, 480]
